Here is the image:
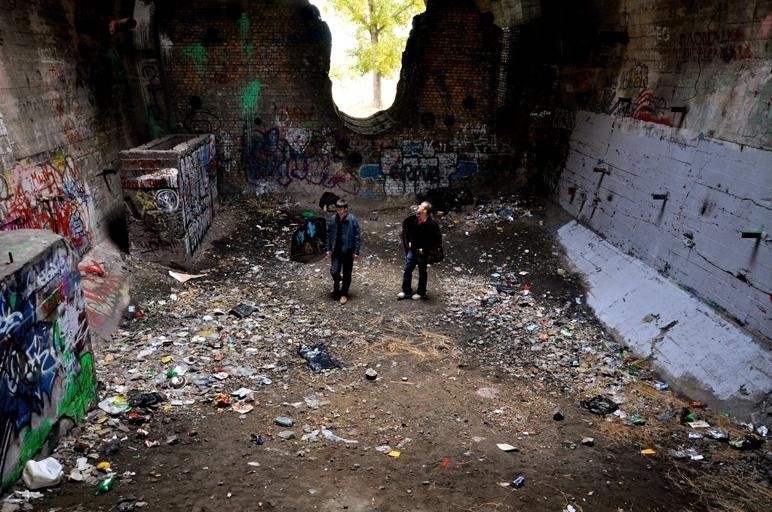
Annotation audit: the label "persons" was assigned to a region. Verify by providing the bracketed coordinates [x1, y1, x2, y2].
[324, 197, 362, 305]
[396, 200, 442, 300]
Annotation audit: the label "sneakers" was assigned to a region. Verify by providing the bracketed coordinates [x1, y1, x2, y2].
[340, 295, 347, 304]
[397, 291, 427, 300]
